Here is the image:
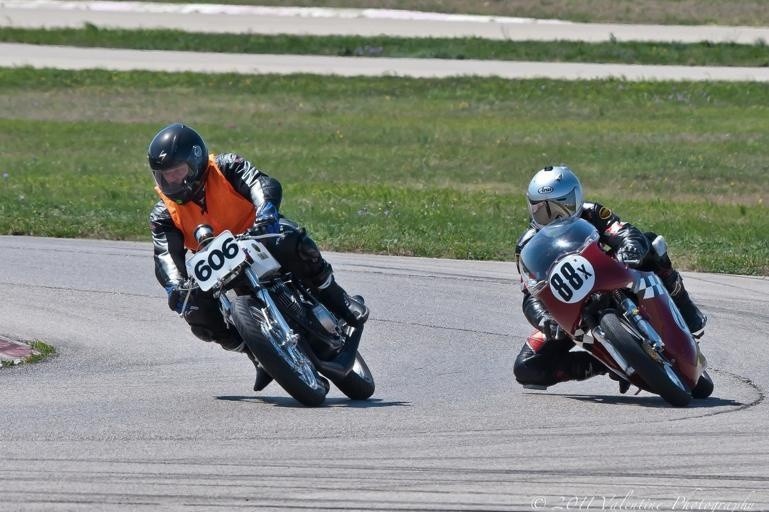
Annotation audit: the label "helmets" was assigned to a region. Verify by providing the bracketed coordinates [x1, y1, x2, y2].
[148, 122, 210, 206]
[524, 165, 585, 238]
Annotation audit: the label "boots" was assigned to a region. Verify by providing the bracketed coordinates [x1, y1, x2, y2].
[573, 352, 629, 394]
[232, 340, 274, 391]
[317, 274, 370, 327]
[663, 268, 707, 334]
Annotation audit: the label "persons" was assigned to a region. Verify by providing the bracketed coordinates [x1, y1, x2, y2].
[514, 166, 706, 393]
[148, 123, 371, 391]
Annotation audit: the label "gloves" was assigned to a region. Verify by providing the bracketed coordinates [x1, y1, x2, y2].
[254, 201, 281, 247]
[164, 284, 195, 316]
[616, 236, 646, 266]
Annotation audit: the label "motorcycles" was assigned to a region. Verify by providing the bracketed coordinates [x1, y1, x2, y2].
[519, 216, 714, 406]
[167, 224, 375, 406]
